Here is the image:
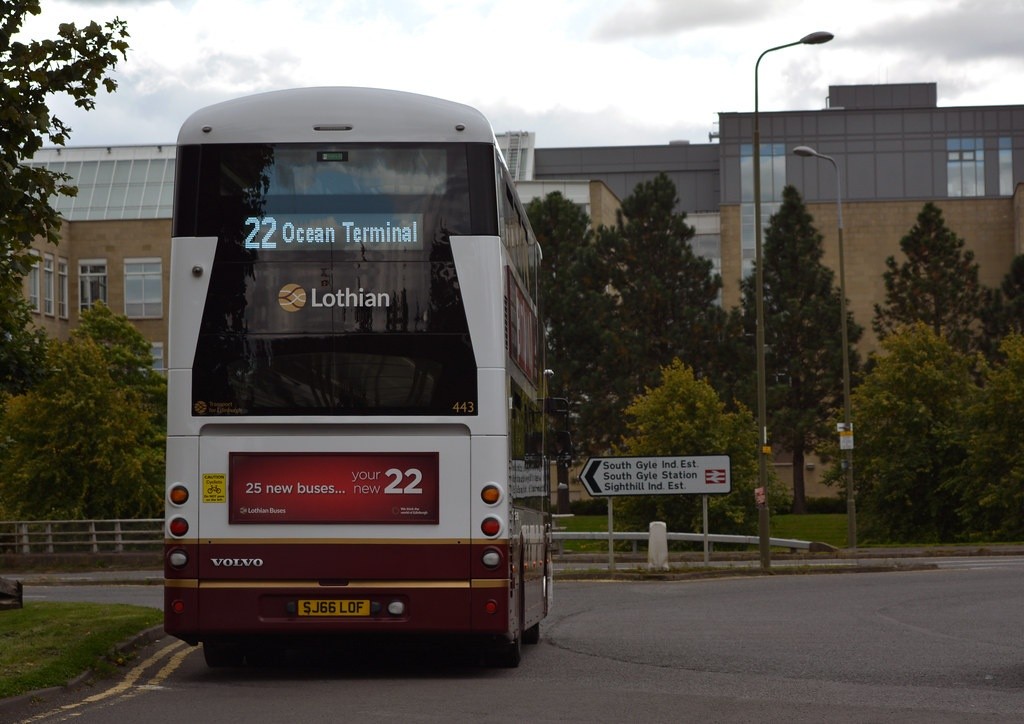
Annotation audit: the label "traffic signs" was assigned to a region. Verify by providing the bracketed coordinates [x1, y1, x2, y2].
[580, 456, 730, 495]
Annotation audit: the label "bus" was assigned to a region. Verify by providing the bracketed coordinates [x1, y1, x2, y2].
[160, 83, 559, 672]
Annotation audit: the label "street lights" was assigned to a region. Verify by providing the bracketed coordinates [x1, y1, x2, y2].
[792, 145, 860, 552]
[755, 31, 835, 571]
